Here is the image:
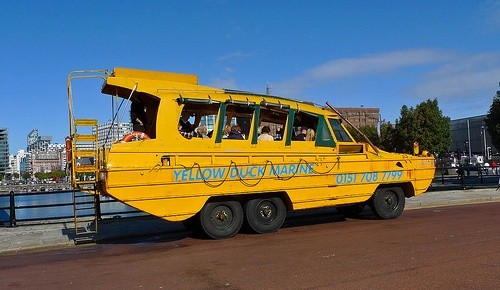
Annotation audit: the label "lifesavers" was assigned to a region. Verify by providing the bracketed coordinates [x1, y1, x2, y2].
[65, 136, 72, 165]
[122, 131, 150, 142]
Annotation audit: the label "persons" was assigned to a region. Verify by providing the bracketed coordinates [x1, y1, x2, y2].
[178, 107, 214, 139]
[221, 125, 250, 140]
[257, 123, 317, 141]
[477, 160, 500, 175]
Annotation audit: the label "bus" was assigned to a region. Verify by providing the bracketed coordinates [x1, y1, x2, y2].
[66, 67, 437, 240]
